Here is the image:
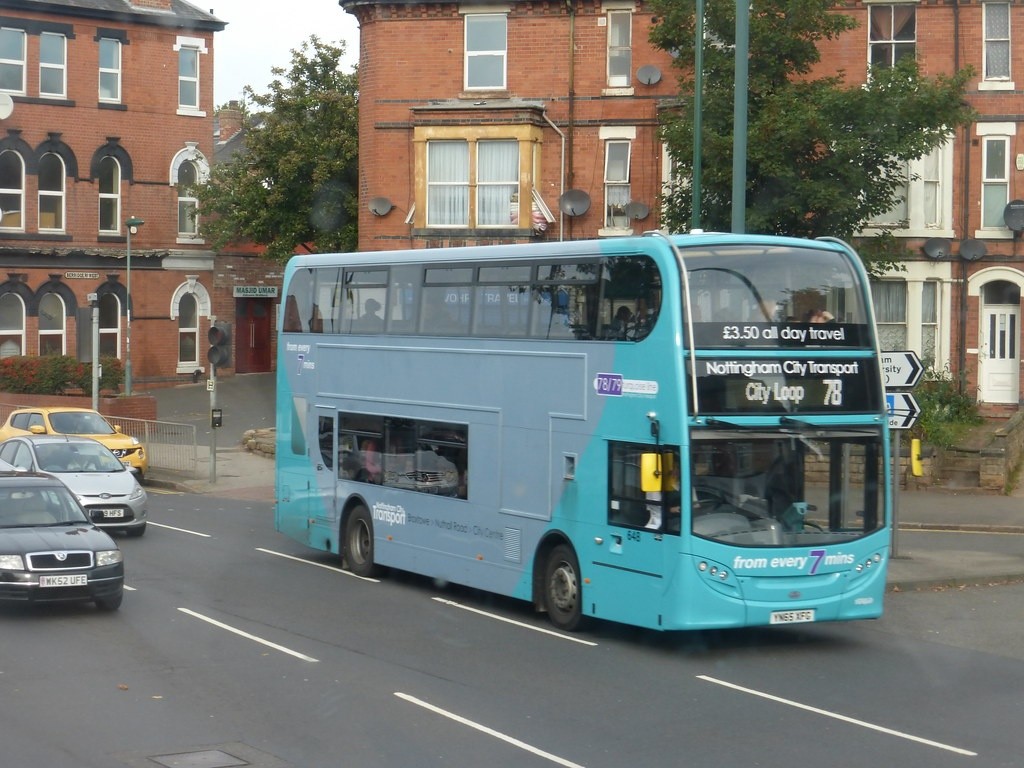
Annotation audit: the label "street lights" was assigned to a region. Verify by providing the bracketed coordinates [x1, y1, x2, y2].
[125, 215, 146, 396]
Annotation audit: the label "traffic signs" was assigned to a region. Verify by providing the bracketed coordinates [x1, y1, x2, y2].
[885, 393, 922, 431]
[878, 351, 925, 390]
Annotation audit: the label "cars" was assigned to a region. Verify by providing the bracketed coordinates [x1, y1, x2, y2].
[0, 406, 148, 487]
[0, 477, 124, 612]
[0, 434, 148, 538]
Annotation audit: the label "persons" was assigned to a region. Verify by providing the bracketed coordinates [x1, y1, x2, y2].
[399, 459, 414, 483]
[73, 418, 83, 433]
[362, 299, 381, 319]
[608, 306, 636, 341]
[68, 453, 96, 470]
[668, 461, 700, 513]
[436, 464, 458, 499]
[803, 307, 836, 323]
[360, 438, 382, 482]
[751, 296, 776, 322]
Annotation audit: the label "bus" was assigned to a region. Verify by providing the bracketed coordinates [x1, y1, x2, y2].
[272, 232, 930, 634]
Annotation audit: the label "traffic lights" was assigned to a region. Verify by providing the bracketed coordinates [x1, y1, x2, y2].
[207, 323, 233, 369]
